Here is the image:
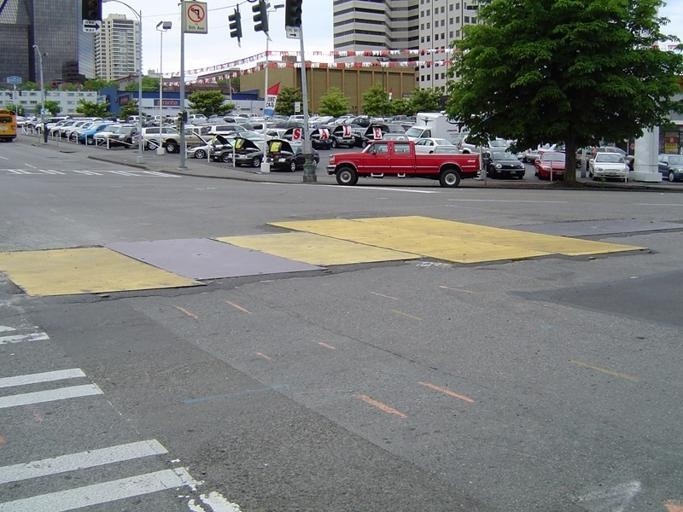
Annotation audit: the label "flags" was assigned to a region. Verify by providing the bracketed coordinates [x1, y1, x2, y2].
[265, 83, 280, 116]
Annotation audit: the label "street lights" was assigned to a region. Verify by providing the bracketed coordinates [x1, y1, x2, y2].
[225, 69, 237, 103]
[257, 2, 284, 174]
[102, 0, 143, 163]
[376, 57, 389, 92]
[31, 44, 49, 112]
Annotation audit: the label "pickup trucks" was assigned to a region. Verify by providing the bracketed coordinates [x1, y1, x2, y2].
[326, 140, 482, 188]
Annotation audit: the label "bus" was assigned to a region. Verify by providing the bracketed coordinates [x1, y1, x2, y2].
[0, 109, 17, 143]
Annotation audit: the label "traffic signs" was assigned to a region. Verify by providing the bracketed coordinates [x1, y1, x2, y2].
[82, 19, 102, 34]
[286, 26, 300, 39]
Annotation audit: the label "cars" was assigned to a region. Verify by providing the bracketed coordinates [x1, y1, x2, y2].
[588, 152, 630, 182]
[658, 153, 683, 182]
[534, 152, 566, 180]
[482, 150, 526, 180]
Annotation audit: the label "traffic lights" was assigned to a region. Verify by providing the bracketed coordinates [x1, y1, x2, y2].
[82, 0, 102, 20]
[252, 0, 269, 32]
[285, 1, 303, 27]
[228, 12, 242, 38]
